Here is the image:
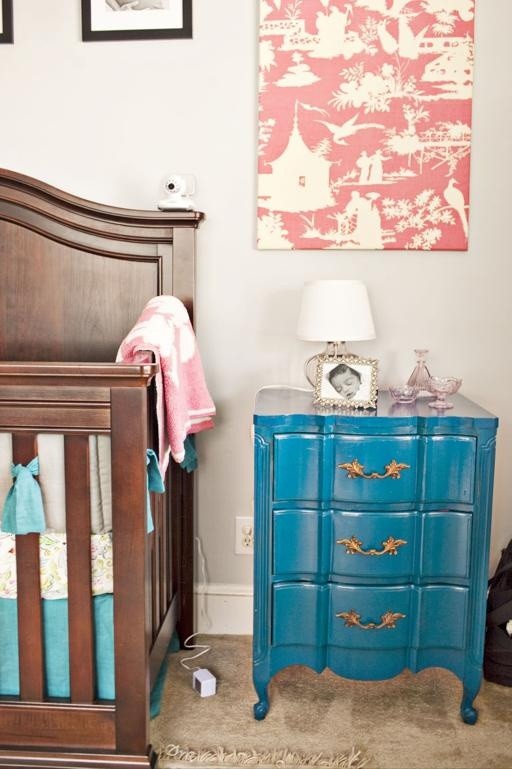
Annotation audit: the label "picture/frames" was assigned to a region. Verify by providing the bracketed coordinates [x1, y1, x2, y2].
[313, 354, 380, 412]
[81, 1, 193, 42]
[2, 0, 16, 45]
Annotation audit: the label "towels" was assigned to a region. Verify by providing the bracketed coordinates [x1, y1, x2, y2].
[117, 293, 215, 478]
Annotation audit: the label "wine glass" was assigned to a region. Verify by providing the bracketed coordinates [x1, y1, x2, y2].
[423, 375, 464, 410]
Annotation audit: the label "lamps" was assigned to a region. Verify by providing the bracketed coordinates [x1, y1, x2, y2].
[300, 273, 373, 394]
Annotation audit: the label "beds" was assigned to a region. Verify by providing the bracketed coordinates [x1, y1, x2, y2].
[1, 164, 204, 768]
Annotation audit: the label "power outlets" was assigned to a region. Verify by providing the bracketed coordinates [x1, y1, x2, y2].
[234, 511, 256, 558]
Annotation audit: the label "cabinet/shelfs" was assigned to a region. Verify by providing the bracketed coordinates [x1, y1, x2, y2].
[250, 388, 500, 726]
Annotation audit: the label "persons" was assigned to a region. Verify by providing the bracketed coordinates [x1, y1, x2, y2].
[327, 363, 361, 400]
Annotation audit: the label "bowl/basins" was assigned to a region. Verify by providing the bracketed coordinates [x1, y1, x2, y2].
[388, 384, 421, 404]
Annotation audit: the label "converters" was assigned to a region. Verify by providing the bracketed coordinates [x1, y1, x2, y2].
[192, 668, 217, 698]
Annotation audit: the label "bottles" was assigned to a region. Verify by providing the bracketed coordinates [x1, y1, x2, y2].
[408, 348, 437, 399]
